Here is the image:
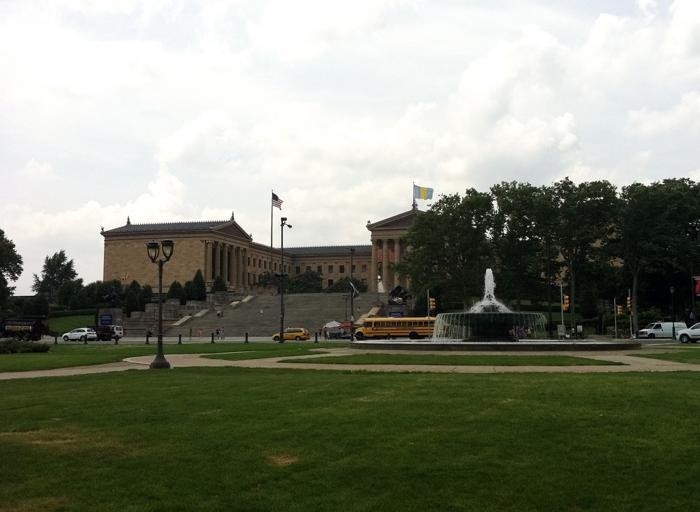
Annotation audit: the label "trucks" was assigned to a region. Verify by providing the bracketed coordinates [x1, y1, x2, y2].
[639, 320, 688, 340]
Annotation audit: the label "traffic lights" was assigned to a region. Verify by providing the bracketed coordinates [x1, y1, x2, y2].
[564, 295, 570, 311]
[626, 296, 632, 309]
[618, 305, 623, 317]
[429, 297, 436, 311]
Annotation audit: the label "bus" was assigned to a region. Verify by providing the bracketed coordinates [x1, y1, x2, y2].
[354, 316, 446, 341]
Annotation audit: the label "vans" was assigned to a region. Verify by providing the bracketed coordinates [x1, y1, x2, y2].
[91, 324, 124, 340]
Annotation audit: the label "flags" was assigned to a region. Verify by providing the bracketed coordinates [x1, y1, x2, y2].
[273, 193, 283, 209]
[414, 183, 433, 200]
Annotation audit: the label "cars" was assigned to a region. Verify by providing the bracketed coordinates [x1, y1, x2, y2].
[271, 326, 311, 342]
[676, 322, 700, 343]
[61, 326, 98, 341]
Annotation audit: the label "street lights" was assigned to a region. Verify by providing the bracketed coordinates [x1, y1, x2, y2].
[142, 239, 176, 370]
[348, 247, 356, 342]
[279, 215, 293, 344]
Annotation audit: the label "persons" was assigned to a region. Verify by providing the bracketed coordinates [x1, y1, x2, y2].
[219, 328, 220, 334]
[216, 328, 221, 340]
[508, 325, 533, 339]
[350, 313, 355, 321]
[222, 326, 225, 337]
[357, 306, 361, 313]
